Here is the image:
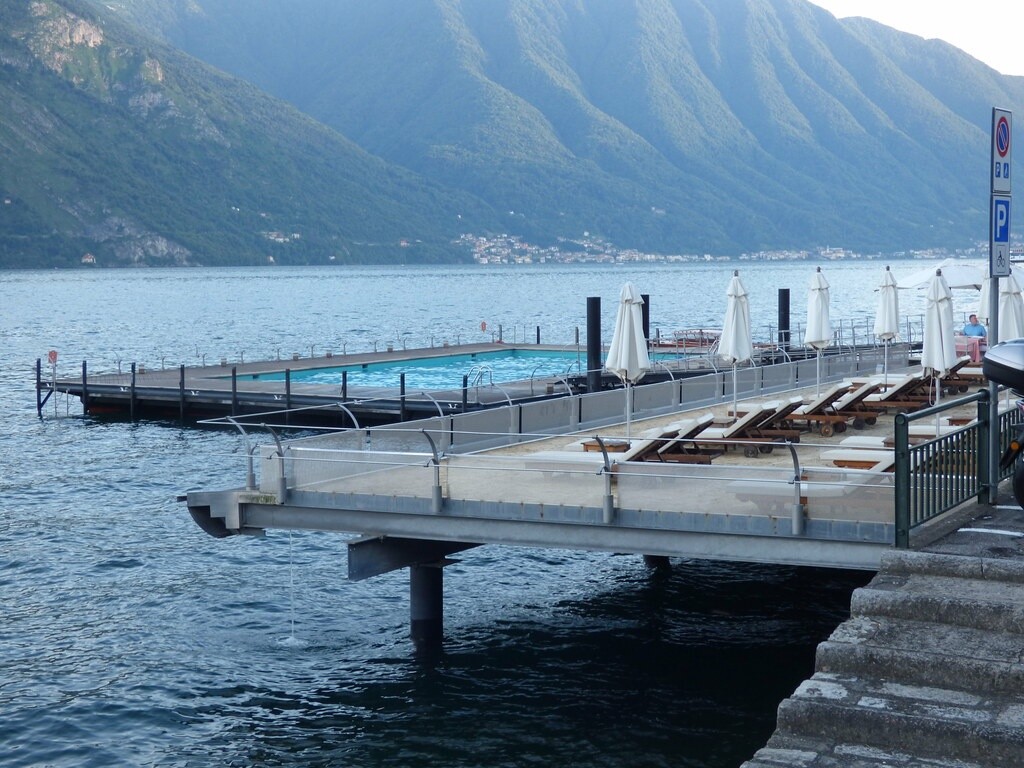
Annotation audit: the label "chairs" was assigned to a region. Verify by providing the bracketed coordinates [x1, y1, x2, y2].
[522, 352, 1024, 522]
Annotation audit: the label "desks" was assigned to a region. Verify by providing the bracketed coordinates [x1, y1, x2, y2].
[710, 417, 736, 428]
[946, 414, 978, 425]
[581, 439, 630, 454]
[883, 436, 926, 448]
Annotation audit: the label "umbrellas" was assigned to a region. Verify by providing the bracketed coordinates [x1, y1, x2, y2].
[998, 269, 1024, 408]
[921, 269, 957, 448]
[606, 281, 651, 444]
[873, 258, 985, 289]
[719, 270, 754, 418]
[806, 267, 832, 399]
[980, 260, 1024, 289]
[964, 315, 987, 337]
[874, 266, 899, 393]
